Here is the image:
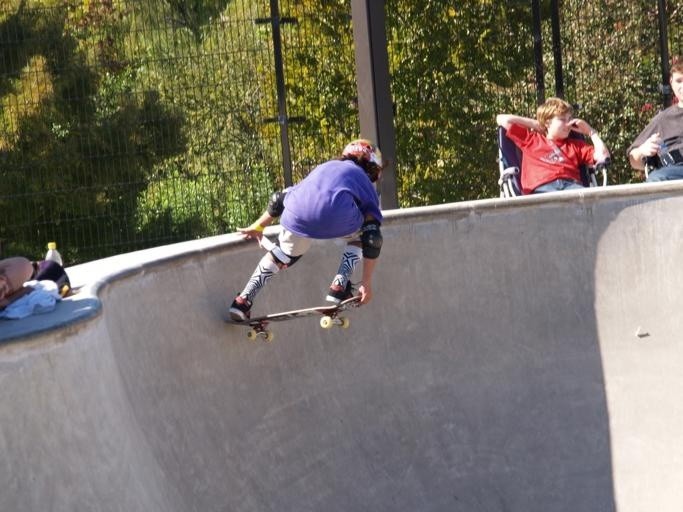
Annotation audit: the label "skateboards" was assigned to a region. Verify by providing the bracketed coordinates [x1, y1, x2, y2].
[224, 296, 364, 341]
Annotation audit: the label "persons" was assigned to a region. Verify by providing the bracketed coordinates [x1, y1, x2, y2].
[497, 97, 611, 194]
[228, 139, 384, 322]
[625, 60, 683, 182]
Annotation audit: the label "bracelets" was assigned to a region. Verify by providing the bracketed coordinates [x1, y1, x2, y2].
[253, 221, 265, 233]
[588, 129, 598, 137]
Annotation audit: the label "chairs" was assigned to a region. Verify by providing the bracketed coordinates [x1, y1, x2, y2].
[496, 118, 608, 198]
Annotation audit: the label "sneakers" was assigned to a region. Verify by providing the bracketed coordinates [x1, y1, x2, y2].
[325, 280, 360, 305]
[228, 292, 253, 322]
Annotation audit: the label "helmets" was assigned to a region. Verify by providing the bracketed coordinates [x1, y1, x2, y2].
[342, 138, 384, 180]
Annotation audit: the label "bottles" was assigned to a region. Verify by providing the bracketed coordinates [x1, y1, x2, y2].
[43, 241, 63, 268]
[655, 132, 675, 169]
